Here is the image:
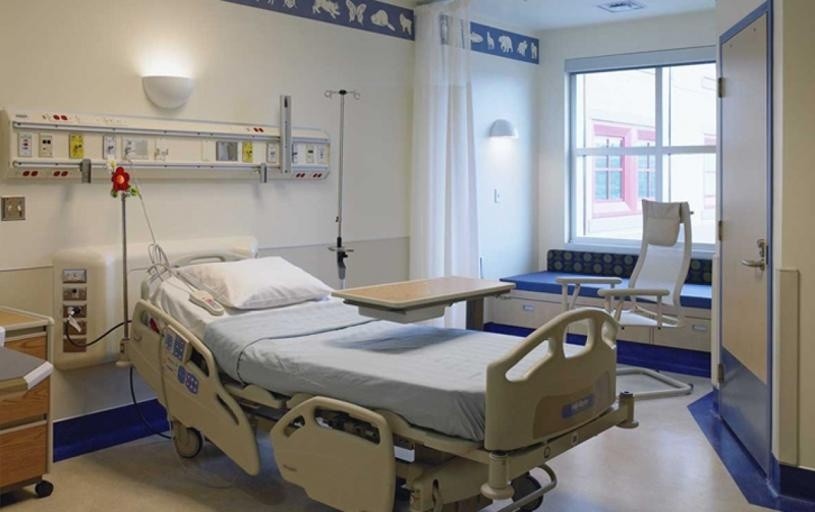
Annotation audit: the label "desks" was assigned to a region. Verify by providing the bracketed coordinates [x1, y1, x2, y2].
[331, 273, 517, 324]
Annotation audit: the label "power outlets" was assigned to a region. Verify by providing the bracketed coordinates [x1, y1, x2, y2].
[63, 303, 87, 319]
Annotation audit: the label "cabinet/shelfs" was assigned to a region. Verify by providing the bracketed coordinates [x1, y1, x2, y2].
[1, 302, 56, 496]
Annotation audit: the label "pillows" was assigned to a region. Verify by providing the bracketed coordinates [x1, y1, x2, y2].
[169, 256, 334, 310]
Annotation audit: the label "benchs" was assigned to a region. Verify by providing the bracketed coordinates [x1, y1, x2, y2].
[491, 248, 711, 352]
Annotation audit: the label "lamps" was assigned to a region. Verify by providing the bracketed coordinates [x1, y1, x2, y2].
[488, 119, 519, 139]
[143, 74, 195, 110]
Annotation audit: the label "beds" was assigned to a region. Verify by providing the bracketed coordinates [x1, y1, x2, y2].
[126, 245, 639, 512]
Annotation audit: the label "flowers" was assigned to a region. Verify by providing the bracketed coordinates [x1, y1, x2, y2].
[108, 159, 140, 199]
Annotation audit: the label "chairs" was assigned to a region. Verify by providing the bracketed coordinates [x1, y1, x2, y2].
[555, 198, 694, 402]
